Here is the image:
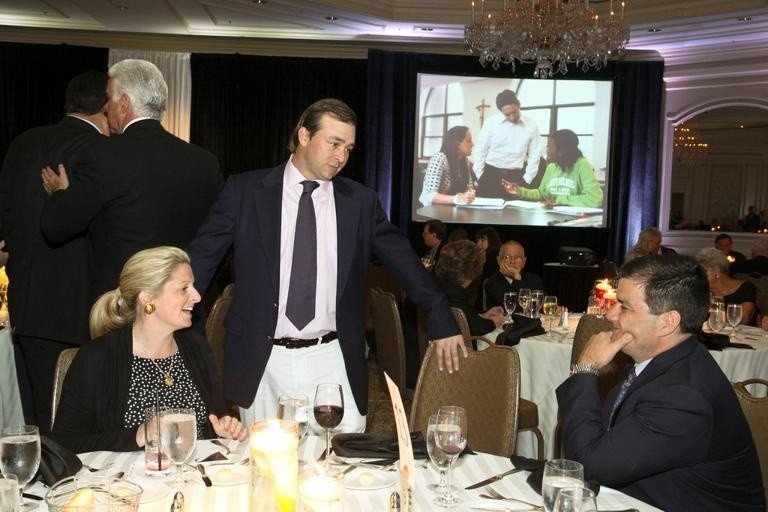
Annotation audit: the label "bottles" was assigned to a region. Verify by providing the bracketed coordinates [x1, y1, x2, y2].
[560, 306, 570, 329]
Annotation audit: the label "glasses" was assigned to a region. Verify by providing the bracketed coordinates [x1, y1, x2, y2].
[501, 253, 524, 261]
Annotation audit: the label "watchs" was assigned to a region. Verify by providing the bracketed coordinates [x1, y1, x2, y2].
[568, 361, 597, 377]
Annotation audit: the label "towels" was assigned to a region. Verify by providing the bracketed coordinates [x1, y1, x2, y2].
[496, 313, 548, 347]
[510, 454, 600, 499]
[697, 332, 756, 352]
[329, 434, 479, 462]
[30, 435, 81, 488]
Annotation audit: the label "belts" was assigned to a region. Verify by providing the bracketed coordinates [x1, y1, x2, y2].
[271, 331, 341, 351]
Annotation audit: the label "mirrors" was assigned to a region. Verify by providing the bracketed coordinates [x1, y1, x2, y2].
[657, 96, 768, 245]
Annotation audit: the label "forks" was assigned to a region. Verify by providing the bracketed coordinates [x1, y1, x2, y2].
[485, 486, 542, 509]
[81, 464, 99, 471]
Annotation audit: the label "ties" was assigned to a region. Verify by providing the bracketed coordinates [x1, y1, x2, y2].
[284, 181, 319, 330]
[605, 368, 639, 434]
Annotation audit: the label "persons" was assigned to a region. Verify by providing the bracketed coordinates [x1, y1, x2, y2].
[0, 70, 110, 436]
[692, 204, 767, 233]
[624, 230, 768, 325]
[182, 97, 469, 462]
[471, 90, 541, 198]
[418, 126, 478, 206]
[501, 129, 603, 208]
[48, 246, 249, 455]
[40, 57, 234, 319]
[552, 256, 768, 511]
[420, 219, 543, 336]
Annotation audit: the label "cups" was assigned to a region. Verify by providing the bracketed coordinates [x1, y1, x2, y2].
[249, 418, 297, 510]
[554, 484, 595, 512]
[297, 467, 344, 511]
[587, 298, 601, 315]
[145, 406, 172, 476]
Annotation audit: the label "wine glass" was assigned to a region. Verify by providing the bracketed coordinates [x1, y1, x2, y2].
[708, 295, 743, 343]
[0, 425, 41, 512]
[278, 392, 309, 466]
[159, 409, 205, 489]
[313, 383, 342, 477]
[540, 457, 584, 511]
[504, 288, 559, 337]
[426, 405, 468, 507]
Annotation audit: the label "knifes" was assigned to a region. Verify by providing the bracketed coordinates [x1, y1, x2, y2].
[465, 465, 529, 490]
[197, 464, 212, 487]
[334, 458, 366, 479]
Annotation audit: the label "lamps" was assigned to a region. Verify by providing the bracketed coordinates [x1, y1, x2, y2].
[671, 124, 711, 163]
[463, 0, 629, 77]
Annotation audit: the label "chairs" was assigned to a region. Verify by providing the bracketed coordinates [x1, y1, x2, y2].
[49, 348, 76, 429]
[203, 300, 228, 417]
[419, 303, 543, 459]
[408, 337, 520, 461]
[726, 377, 768, 488]
[366, 286, 415, 443]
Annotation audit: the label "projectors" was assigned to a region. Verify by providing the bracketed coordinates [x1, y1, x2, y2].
[556, 245, 598, 266]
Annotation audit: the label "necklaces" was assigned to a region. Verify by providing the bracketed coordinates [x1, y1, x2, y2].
[142, 337, 177, 386]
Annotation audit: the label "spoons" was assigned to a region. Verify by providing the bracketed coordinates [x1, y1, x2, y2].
[48, 471, 125, 498]
